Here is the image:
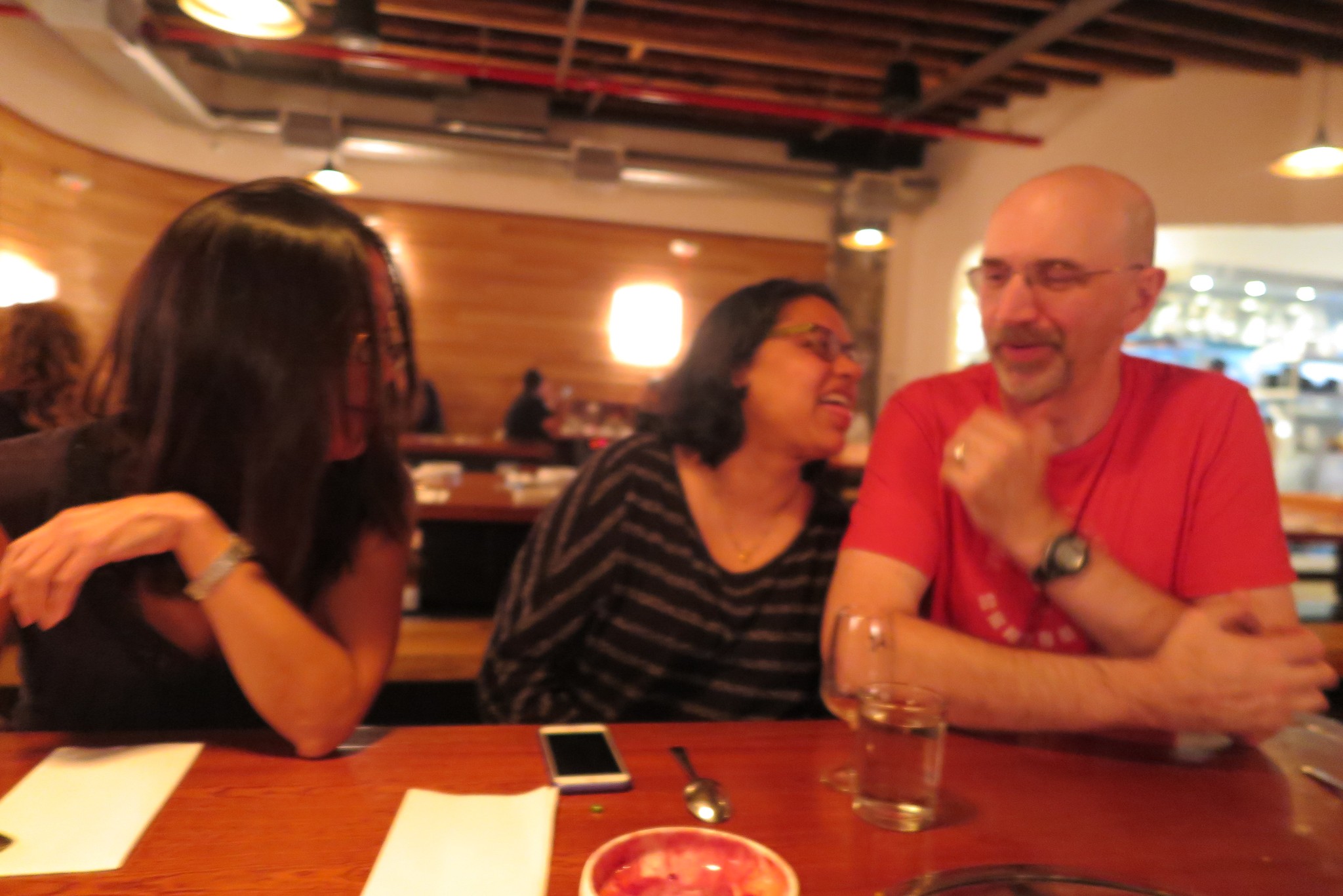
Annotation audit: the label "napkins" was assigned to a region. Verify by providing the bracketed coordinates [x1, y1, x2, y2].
[0, 744, 205, 877]
[358, 784, 560, 896]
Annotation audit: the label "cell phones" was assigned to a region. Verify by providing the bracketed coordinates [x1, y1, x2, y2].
[539, 724, 631, 793]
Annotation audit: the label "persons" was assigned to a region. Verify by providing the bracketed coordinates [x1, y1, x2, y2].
[474, 277, 931, 733]
[820, 163, 1339, 763]
[502, 370, 551, 441]
[0, 301, 97, 440]
[0, 176, 415, 760]
[401, 382, 444, 465]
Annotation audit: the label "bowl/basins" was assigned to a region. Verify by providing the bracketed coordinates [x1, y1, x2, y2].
[578, 827, 799, 896]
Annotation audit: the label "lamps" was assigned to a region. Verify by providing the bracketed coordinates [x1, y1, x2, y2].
[310, 163, 358, 195]
[838, 175, 937, 254]
[177, 0, 313, 40]
[1274, 130, 1343, 182]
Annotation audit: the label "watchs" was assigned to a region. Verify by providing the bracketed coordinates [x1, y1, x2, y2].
[1032, 529, 1088, 583]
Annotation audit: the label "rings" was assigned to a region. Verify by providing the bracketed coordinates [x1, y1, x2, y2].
[954, 445, 964, 458]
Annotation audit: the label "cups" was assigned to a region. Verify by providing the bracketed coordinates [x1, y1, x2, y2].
[850, 681, 946, 832]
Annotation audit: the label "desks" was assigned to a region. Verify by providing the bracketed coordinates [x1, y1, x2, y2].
[0, 433, 1343, 896]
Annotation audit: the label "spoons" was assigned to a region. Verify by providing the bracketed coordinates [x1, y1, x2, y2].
[669, 745, 732, 823]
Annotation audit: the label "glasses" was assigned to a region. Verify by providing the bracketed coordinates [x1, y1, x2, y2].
[759, 324, 861, 365]
[966, 261, 1143, 297]
[346, 321, 405, 364]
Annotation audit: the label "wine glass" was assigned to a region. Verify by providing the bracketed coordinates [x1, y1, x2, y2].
[821, 609, 897, 793]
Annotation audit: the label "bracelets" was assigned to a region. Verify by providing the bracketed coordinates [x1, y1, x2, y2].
[183, 532, 256, 600]
